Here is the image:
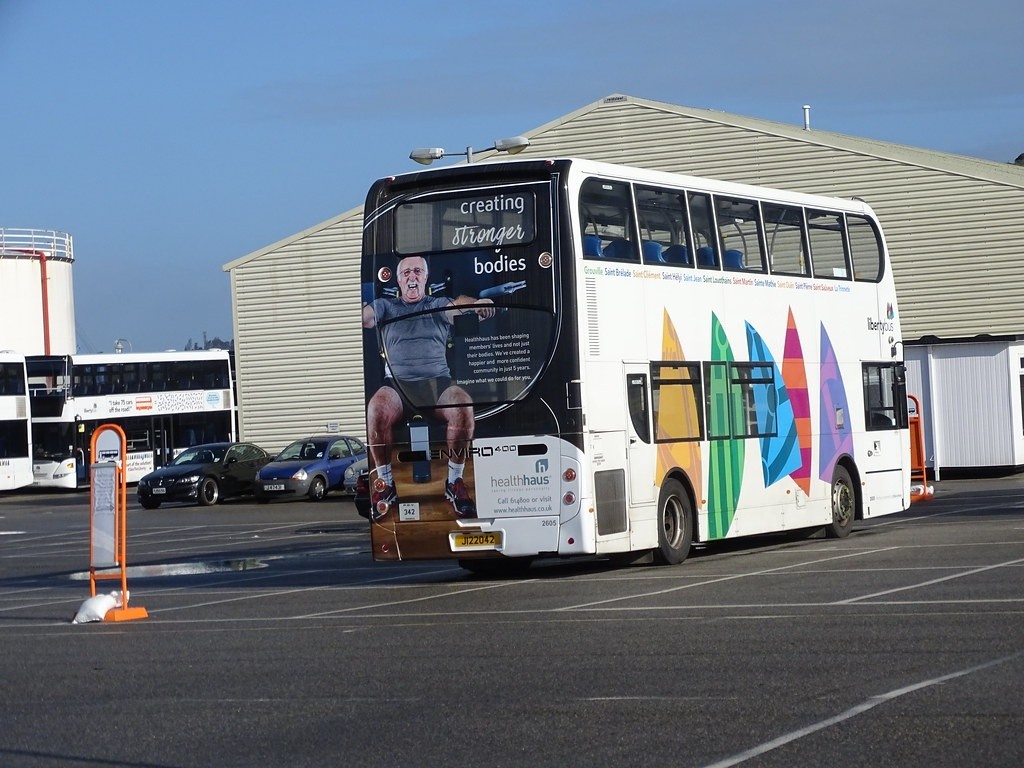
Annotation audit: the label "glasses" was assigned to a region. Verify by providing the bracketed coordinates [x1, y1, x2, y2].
[399, 267, 426, 277]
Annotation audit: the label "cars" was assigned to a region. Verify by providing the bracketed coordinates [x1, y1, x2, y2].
[136, 442, 278, 510]
[343, 457, 368, 494]
[354, 472, 371, 520]
[254, 435, 367, 504]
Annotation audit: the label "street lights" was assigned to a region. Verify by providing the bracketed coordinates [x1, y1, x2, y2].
[409, 136, 531, 247]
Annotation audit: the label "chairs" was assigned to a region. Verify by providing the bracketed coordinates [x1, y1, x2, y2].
[221, 450, 238, 463]
[181, 429, 197, 448]
[4, 381, 19, 393]
[866, 411, 892, 428]
[243, 449, 257, 460]
[580, 231, 746, 272]
[331, 446, 345, 458]
[205, 431, 217, 444]
[198, 449, 214, 463]
[73, 373, 232, 397]
[305, 448, 318, 458]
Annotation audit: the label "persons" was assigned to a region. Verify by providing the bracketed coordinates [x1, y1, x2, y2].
[362, 257, 495, 520]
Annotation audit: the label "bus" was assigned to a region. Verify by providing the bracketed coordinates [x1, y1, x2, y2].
[359, 157, 912, 577]
[24, 348, 236, 489]
[0, 350, 35, 490]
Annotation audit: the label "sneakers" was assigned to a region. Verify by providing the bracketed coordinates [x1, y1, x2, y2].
[445, 478, 475, 516]
[372, 480, 398, 519]
